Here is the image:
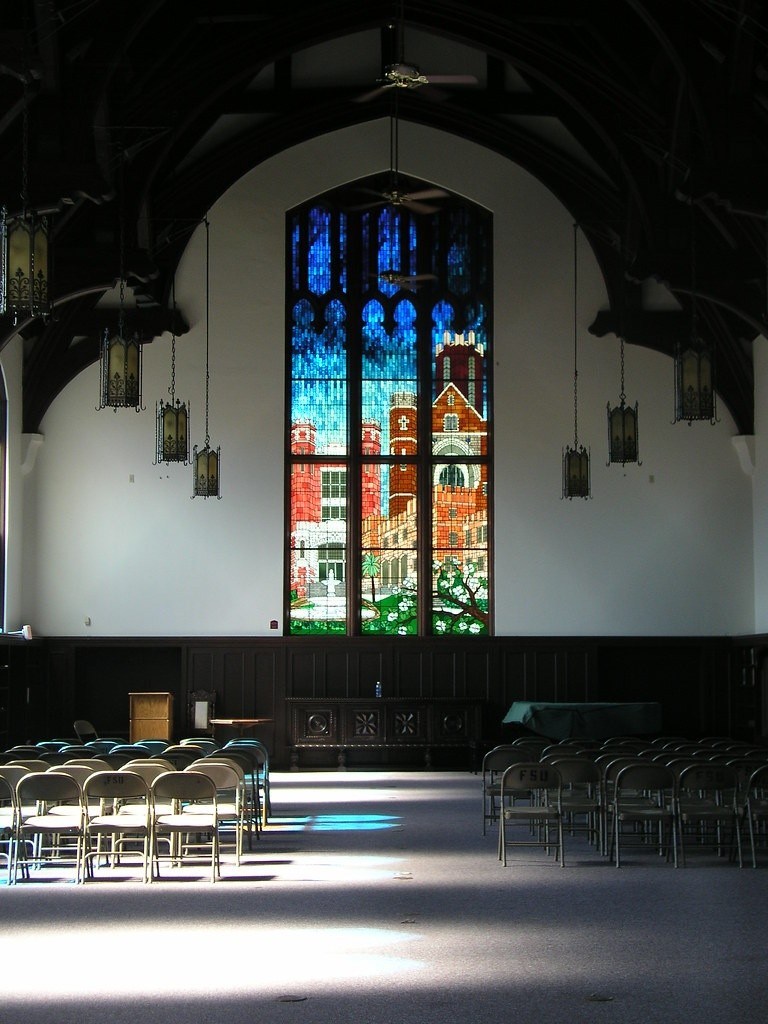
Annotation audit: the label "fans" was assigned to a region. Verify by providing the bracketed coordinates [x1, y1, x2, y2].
[352, 1, 479, 292]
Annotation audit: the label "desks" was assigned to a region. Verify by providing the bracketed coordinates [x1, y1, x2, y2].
[502, 700, 663, 740]
[210, 719, 273, 737]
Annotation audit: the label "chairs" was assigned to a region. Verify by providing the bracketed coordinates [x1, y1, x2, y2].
[482, 734, 768, 869]
[0, 719, 275, 886]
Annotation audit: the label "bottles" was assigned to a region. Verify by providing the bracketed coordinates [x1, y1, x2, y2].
[376, 682, 382, 698]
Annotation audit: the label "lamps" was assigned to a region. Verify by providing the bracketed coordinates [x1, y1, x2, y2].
[0, 0, 220, 499]
[563, 0, 717, 502]
